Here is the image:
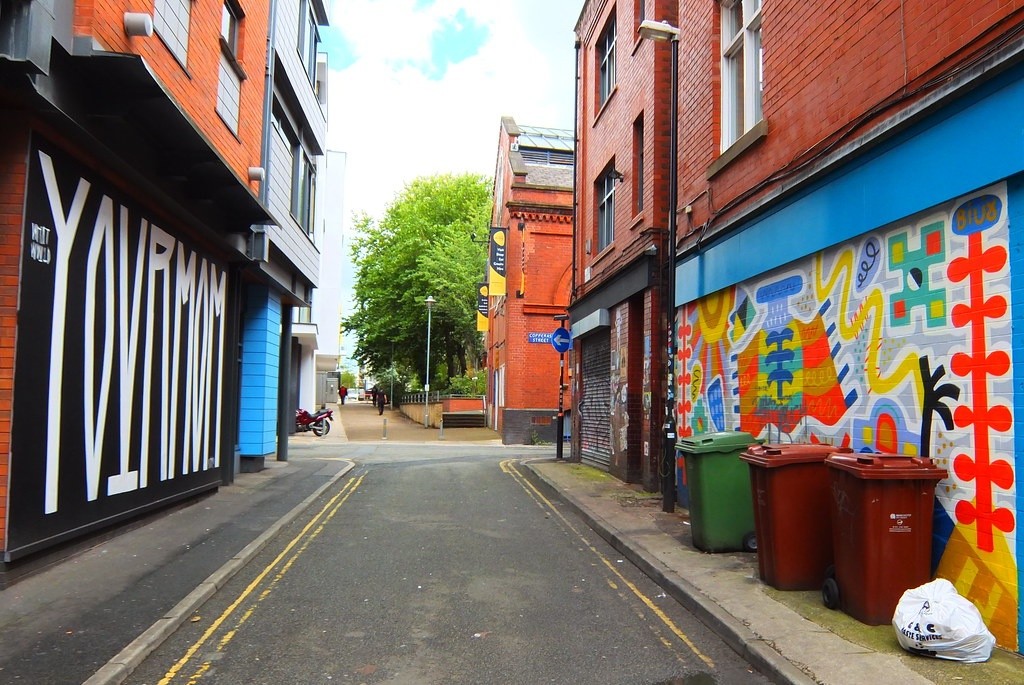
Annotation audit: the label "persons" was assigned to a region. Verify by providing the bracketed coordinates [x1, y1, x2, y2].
[371, 384, 388, 416]
[338, 385, 347, 405]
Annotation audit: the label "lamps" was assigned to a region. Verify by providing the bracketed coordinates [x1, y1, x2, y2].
[608, 170, 623, 182]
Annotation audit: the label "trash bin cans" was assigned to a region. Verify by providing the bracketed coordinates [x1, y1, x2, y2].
[823, 451, 949, 627]
[739, 443, 854, 591]
[674, 431, 766, 553]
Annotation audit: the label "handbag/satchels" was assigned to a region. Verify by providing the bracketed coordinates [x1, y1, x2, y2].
[891, 578, 996, 663]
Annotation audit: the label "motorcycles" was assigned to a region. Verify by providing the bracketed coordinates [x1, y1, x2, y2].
[295, 408, 334, 437]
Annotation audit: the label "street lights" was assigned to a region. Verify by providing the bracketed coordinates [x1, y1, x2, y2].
[423, 296, 437, 428]
[637, 20, 682, 512]
